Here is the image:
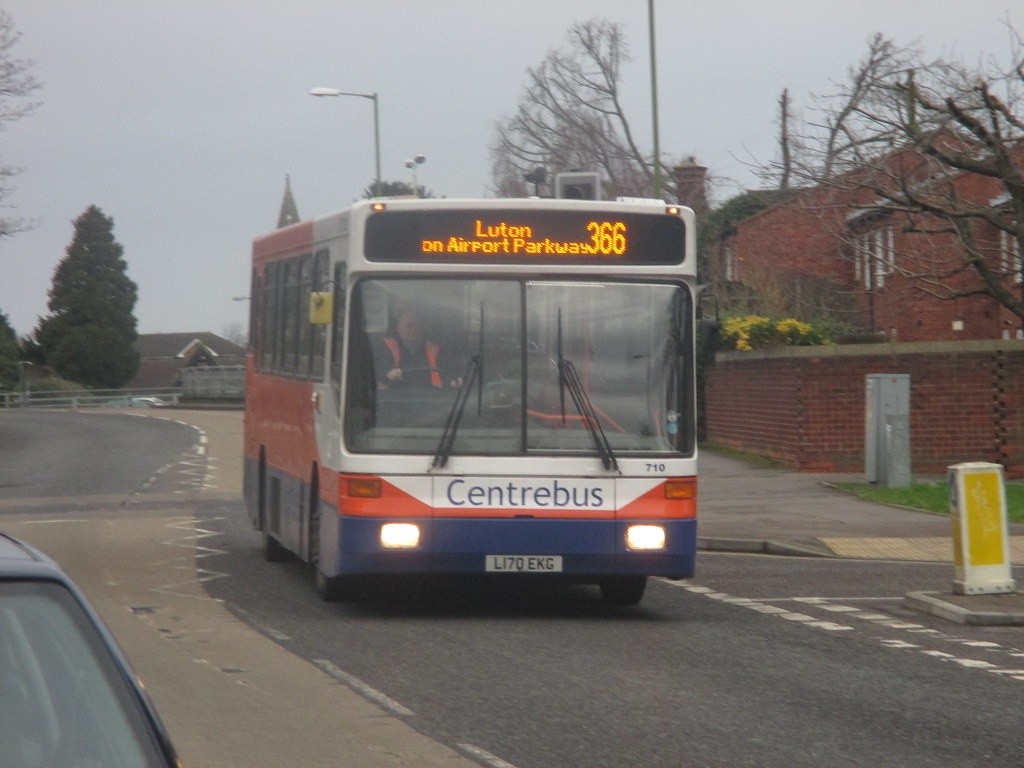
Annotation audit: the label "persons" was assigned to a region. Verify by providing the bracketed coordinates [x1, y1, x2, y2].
[372, 307, 464, 395]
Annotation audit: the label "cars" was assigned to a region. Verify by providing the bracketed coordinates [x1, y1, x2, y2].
[105, 396, 169, 407]
[1, 531, 179, 767]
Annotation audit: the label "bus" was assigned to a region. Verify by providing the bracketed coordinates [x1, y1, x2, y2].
[243, 196, 700, 604]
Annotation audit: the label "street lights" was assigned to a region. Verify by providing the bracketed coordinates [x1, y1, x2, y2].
[309, 87, 381, 200]
